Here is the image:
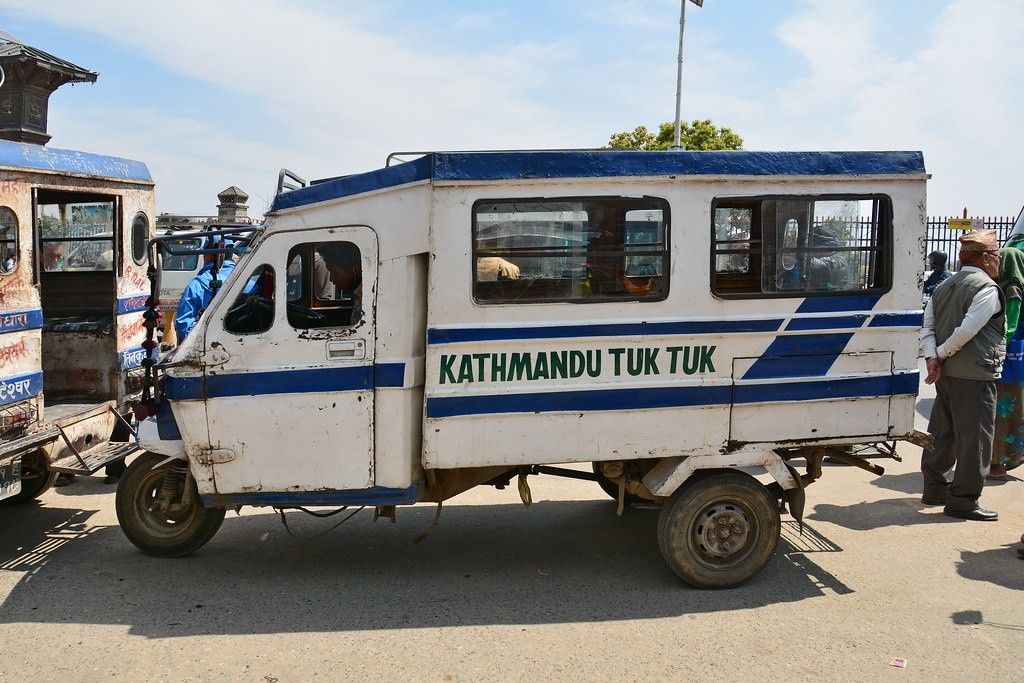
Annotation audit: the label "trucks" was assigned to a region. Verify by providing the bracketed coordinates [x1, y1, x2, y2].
[114, 142, 937, 592]
[0, 137, 164, 512]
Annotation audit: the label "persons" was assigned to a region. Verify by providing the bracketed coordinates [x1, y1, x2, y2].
[924, 250, 953, 294]
[805, 224, 850, 291]
[40, 241, 64, 269]
[987, 246, 1024, 481]
[316, 242, 362, 324]
[920, 230, 1007, 520]
[176, 231, 260, 340]
[475, 224, 521, 281]
[3, 254, 17, 270]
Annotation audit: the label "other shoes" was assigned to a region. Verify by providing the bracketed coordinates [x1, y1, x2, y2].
[54, 474, 75, 486]
[103, 477, 120, 483]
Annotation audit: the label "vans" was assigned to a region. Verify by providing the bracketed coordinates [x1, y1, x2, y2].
[55, 227, 252, 333]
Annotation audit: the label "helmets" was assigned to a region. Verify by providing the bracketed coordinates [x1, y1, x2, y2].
[812, 225, 839, 254]
[928, 250, 947, 271]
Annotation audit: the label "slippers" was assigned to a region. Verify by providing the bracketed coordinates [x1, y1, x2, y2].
[986, 472, 1007, 480]
[1005, 456, 1024, 471]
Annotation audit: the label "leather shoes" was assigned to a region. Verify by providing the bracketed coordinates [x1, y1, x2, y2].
[944, 503, 999, 521]
[921, 482, 953, 505]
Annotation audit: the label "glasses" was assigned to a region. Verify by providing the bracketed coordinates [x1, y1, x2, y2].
[986, 251, 1003, 260]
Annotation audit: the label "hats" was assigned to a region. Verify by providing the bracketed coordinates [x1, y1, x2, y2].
[959, 229, 999, 251]
[781, 255, 795, 271]
[196, 234, 234, 254]
